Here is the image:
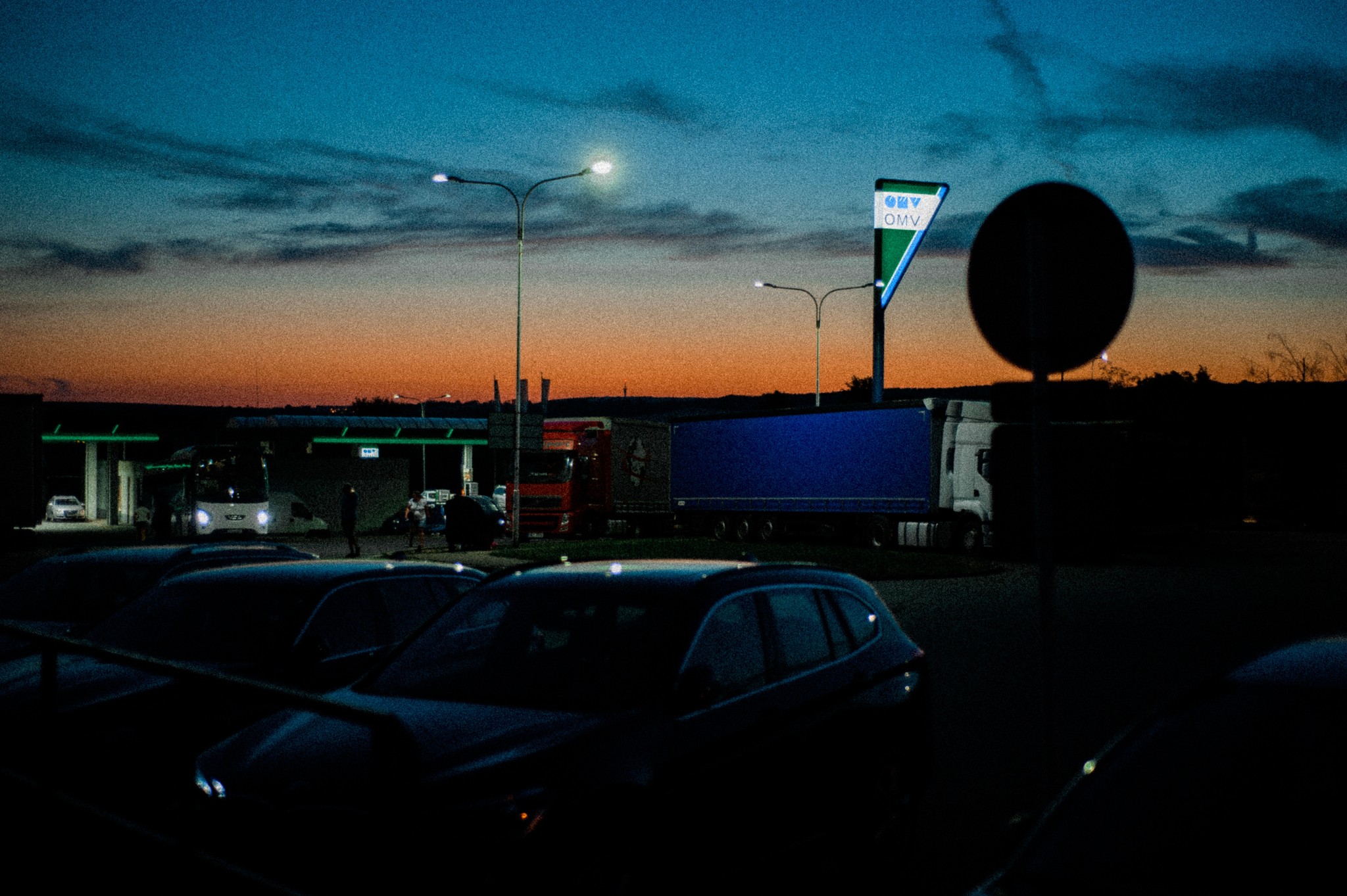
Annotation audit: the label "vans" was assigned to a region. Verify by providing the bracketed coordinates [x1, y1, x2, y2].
[266, 492, 330, 540]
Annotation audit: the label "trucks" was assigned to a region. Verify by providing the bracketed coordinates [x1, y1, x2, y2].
[671, 398, 1014, 576]
[504, 417, 674, 538]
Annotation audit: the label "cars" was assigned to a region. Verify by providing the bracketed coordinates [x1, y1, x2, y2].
[1, 538, 314, 669]
[0, 556, 486, 880]
[945, 638, 1347, 896]
[176, 555, 945, 895]
[44, 494, 87, 524]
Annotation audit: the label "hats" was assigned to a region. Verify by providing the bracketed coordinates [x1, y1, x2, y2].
[413, 491, 422, 498]
[341, 482, 352, 489]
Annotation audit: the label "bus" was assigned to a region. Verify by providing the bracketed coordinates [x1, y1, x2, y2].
[169, 444, 271, 538]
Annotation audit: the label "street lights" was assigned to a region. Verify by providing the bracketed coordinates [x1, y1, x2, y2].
[764, 284, 876, 415]
[430, 158, 612, 558]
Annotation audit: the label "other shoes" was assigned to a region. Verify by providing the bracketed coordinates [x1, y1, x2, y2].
[346, 547, 361, 558]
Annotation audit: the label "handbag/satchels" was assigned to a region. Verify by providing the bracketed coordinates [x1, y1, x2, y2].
[407, 509, 415, 520]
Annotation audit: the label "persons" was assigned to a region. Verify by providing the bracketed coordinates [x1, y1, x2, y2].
[404, 491, 430, 548]
[339, 482, 361, 558]
[133, 501, 151, 541]
